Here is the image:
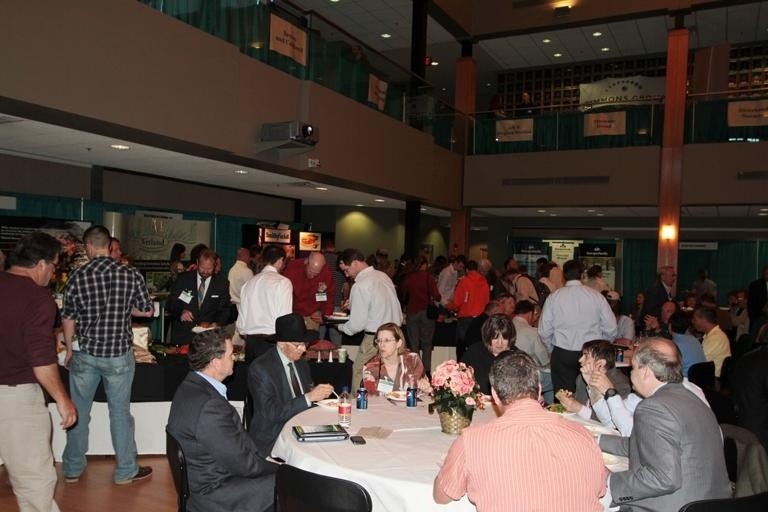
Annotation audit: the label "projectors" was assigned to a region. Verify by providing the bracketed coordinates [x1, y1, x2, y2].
[262, 119, 321, 145]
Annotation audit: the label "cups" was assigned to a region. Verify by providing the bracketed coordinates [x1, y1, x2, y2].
[338, 348, 346, 362]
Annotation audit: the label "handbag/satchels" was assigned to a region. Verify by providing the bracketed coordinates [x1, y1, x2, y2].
[426, 304, 438, 320]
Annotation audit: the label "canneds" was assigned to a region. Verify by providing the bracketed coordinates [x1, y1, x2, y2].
[357, 388, 368, 410]
[615, 349, 624, 362]
[406, 387, 417, 407]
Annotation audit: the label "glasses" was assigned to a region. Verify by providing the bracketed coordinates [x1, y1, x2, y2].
[290, 342, 309, 348]
[374, 338, 395, 343]
[51, 262, 60, 269]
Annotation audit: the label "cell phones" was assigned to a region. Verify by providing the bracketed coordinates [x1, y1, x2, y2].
[351, 436, 366, 444]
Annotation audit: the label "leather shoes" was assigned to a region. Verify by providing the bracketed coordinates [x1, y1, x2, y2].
[115, 466, 152, 485]
[65, 476, 80, 483]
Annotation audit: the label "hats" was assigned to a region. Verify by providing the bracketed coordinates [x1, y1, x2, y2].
[264, 313, 319, 343]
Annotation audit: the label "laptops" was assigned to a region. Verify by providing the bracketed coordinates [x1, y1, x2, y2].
[293, 422, 349, 442]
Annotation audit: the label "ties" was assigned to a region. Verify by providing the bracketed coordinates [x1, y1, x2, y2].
[197, 278, 206, 306]
[287, 363, 300, 397]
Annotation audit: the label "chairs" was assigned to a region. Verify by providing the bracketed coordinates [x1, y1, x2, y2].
[679, 493, 766, 509]
[720, 423, 763, 493]
[241, 390, 252, 433]
[274, 464, 372, 511]
[165, 425, 190, 511]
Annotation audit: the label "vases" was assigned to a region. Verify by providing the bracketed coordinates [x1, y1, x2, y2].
[439, 406, 471, 435]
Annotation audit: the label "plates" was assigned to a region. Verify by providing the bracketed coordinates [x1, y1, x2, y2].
[327, 313, 350, 320]
[314, 399, 339, 410]
[386, 390, 408, 402]
[598, 450, 621, 468]
[553, 411, 582, 416]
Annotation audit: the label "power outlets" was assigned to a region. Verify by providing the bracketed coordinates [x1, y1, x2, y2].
[309, 158, 322, 169]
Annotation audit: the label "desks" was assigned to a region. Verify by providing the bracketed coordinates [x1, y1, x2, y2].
[48, 359, 353, 463]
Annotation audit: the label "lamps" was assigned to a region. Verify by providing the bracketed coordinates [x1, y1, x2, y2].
[659, 224, 677, 286]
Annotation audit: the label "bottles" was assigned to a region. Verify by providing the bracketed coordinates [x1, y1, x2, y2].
[317, 350, 322, 362]
[633, 337, 642, 350]
[338, 387, 350, 427]
[329, 349, 333, 362]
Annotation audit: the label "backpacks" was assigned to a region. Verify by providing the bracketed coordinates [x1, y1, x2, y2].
[514, 274, 550, 309]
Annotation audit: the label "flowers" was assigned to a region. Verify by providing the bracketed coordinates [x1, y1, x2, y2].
[49, 233, 88, 290]
[430, 360, 482, 409]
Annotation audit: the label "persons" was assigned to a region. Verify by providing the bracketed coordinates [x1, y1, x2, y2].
[296, 15, 308, 30]
[352, 45, 368, 63]
[515, 92, 538, 116]
[488, 95, 508, 120]
[0, 226, 768, 512]
